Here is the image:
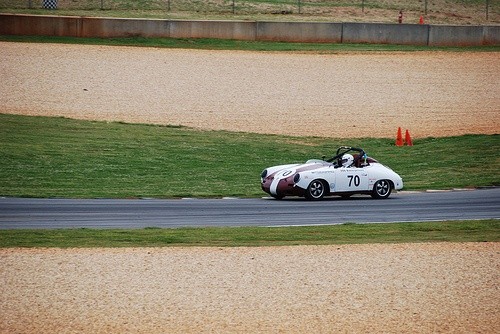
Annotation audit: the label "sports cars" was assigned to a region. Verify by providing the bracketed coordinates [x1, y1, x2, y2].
[260, 145, 404, 202]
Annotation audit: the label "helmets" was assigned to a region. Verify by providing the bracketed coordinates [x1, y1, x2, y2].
[341, 154, 354, 167]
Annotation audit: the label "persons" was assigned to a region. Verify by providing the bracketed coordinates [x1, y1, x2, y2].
[337, 154, 357, 168]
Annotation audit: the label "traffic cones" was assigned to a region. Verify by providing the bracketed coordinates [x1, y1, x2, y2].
[418, 15, 425, 24]
[403, 129, 413, 147]
[394, 127, 404, 147]
